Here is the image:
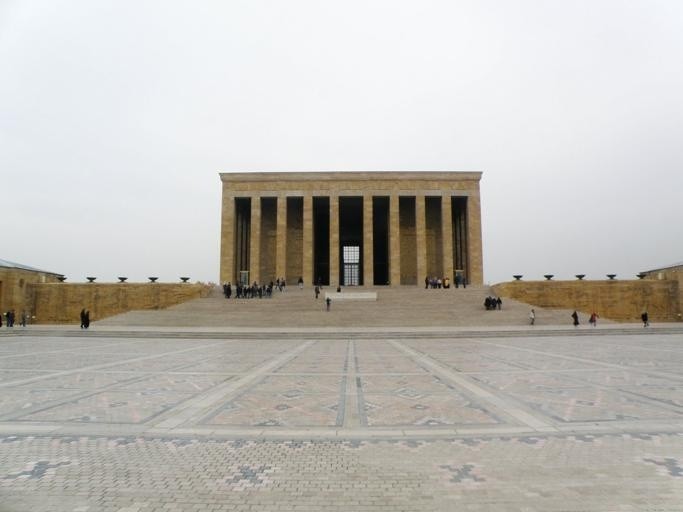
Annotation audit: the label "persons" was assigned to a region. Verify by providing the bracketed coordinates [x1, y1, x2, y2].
[79, 307, 87, 328]
[85, 310, 90, 328]
[530, 308, 535, 325]
[18, 309, 27, 326]
[424, 275, 466, 290]
[314, 284, 320, 299]
[336, 284, 341, 293]
[222, 275, 289, 300]
[570, 310, 579, 326]
[297, 276, 304, 291]
[5, 309, 10, 326]
[324, 296, 333, 311]
[641, 311, 649, 328]
[8, 309, 15, 327]
[483, 295, 502, 311]
[589, 311, 600, 325]
